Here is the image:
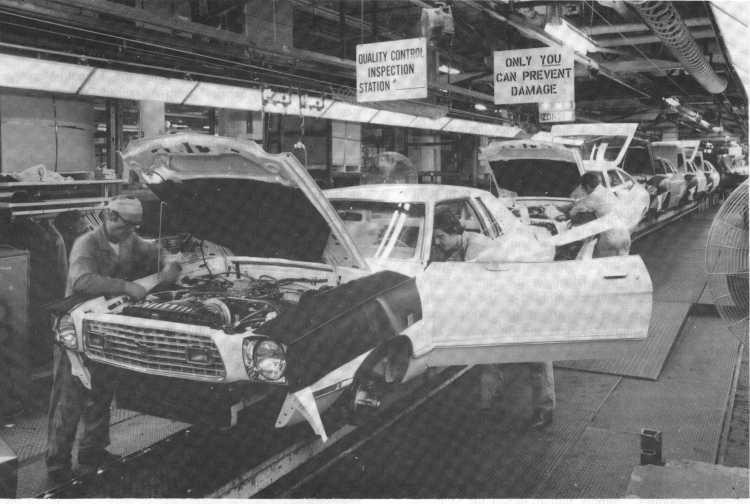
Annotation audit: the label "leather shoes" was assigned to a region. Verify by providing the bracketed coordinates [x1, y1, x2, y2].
[526, 410, 552, 431]
[475, 410, 496, 439]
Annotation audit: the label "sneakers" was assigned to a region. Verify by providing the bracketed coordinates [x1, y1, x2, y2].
[48, 450, 121, 482]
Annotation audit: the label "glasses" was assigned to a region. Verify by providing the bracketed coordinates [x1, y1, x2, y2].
[112, 210, 143, 231]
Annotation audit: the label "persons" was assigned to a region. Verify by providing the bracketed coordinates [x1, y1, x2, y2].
[46, 197, 188, 487]
[433, 211, 557, 431]
[556, 173, 630, 258]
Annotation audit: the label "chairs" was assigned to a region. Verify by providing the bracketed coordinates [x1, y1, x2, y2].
[379, 225, 479, 261]
